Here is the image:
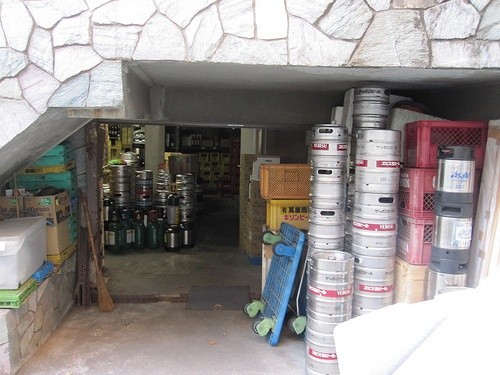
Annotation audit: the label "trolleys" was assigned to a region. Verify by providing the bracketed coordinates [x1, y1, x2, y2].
[242, 221, 308, 348]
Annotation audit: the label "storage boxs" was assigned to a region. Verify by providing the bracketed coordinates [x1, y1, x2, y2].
[69, 186, 82, 243]
[0, 277, 39, 309]
[258, 161, 310, 200]
[8, 138, 76, 191]
[45, 218, 70, 255]
[386, 105, 499, 303]
[0, 193, 23, 220]
[239, 152, 281, 265]
[48, 238, 78, 265]
[17, 190, 73, 225]
[33, 262, 54, 281]
[0, 214, 45, 290]
[265, 199, 309, 231]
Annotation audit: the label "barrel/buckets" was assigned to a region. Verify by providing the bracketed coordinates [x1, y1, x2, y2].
[424, 145, 476, 298]
[102, 143, 199, 253]
[303, 84, 403, 375]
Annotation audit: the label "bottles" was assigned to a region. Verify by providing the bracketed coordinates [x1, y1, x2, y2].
[108, 124, 119, 137]
